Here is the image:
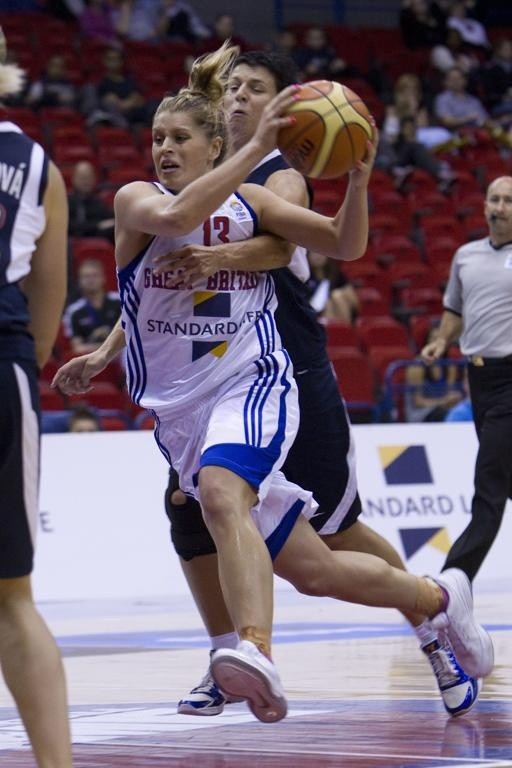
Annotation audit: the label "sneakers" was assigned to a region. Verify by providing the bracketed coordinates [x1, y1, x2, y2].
[177, 637, 288, 722]
[420, 567, 494, 716]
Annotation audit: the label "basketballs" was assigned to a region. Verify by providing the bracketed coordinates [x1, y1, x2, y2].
[275, 80, 375, 179]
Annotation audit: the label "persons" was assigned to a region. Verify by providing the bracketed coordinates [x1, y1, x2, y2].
[421, 176, 511, 585]
[275, 2, 512, 195]
[62, 258, 122, 353]
[68, 401, 100, 430]
[0, 119, 71, 768]
[407, 322, 473, 422]
[113, 38, 494, 723]
[67, 160, 115, 245]
[49, 51, 480, 718]
[0, 32, 157, 129]
[306, 248, 360, 324]
[65, 0, 254, 54]
